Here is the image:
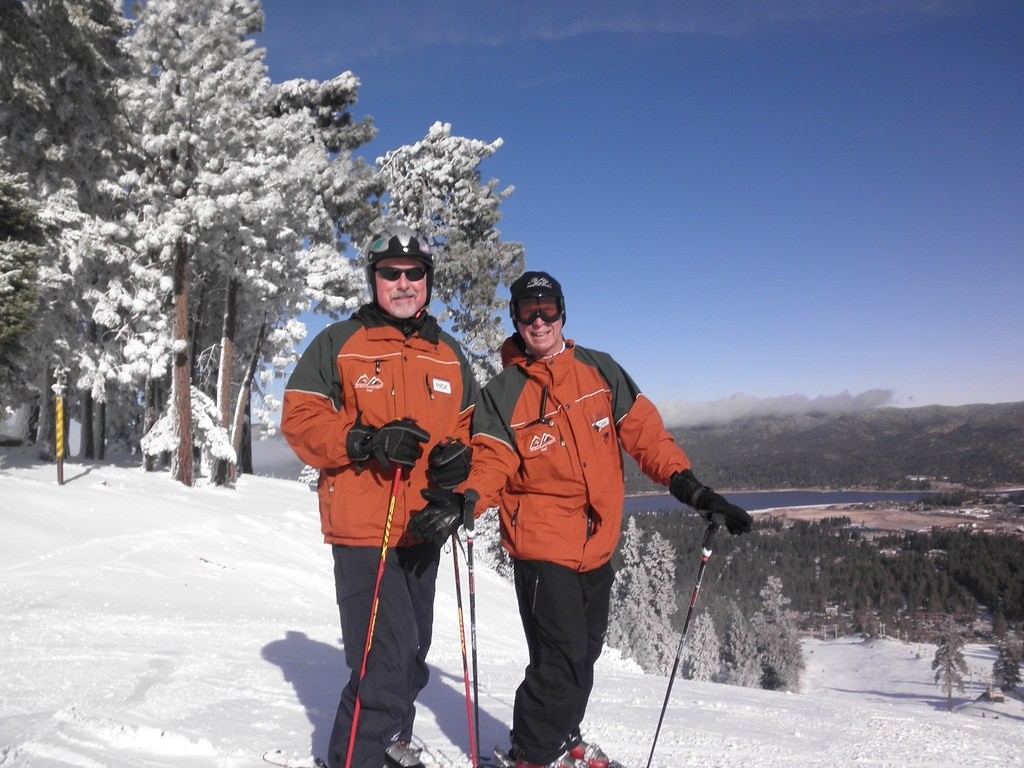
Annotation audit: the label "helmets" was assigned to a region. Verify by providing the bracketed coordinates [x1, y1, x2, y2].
[509, 271, 566, 327]
[363, 225, 434, 306]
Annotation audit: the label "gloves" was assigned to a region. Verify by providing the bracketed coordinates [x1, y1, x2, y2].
[406, 489, 480, 552]
[346, 409, 430, 481]
[425, 436, 472, 491]
[665, 467, 753, 537]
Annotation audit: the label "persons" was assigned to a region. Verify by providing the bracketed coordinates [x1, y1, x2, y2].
[279, 223, 481, 768]
[406, 272, 753, 768]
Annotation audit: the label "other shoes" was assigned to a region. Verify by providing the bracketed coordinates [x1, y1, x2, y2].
[384, 741, 425, 768]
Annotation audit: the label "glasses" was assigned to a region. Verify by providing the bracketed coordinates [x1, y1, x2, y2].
[515, 295, 565, 326]
[374, 267, 428, 281]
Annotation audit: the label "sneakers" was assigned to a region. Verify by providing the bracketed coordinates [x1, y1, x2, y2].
[566, 733, 626, 768]
[493, 730, 591, 768]
